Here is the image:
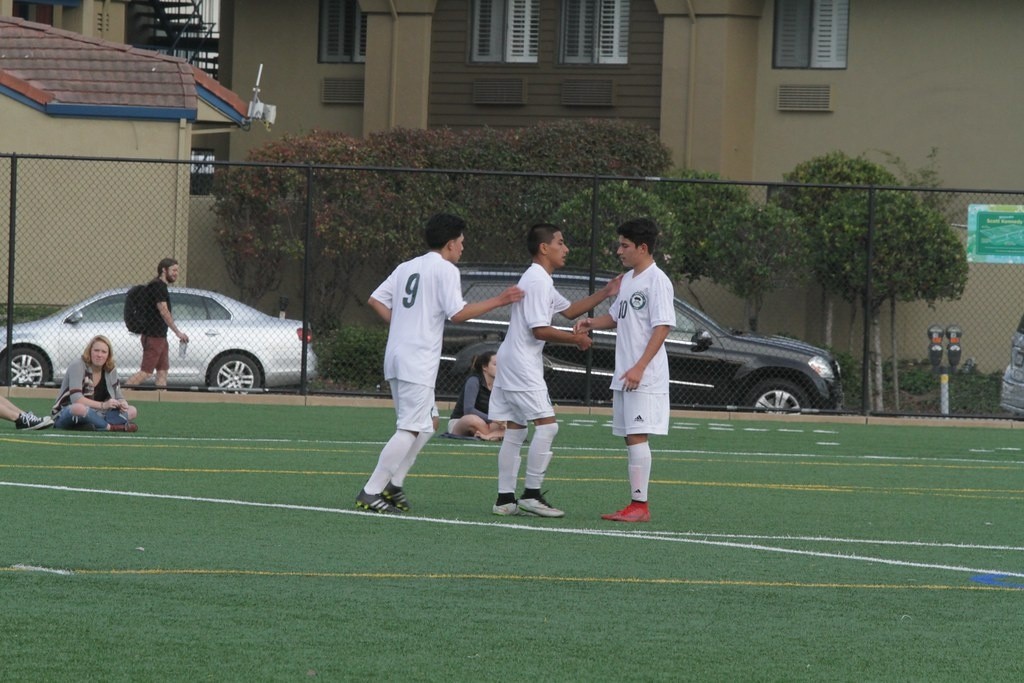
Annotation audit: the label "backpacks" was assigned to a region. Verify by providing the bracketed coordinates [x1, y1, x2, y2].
[124, 278, 160, 334]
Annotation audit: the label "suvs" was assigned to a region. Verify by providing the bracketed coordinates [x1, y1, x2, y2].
[435, 262, 847, 416]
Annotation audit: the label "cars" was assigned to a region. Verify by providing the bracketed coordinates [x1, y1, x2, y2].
[0, 288, 318, 395]
[991, 312, 1024, 417]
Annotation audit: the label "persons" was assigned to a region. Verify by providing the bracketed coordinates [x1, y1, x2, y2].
[124, 258, 189, 391]
[51, 335, 138, 432]
[572, 219, 678, 522]
[0, 396, 54, 431]
[354, 213, 524, 514]
[487, 224, 624, 518]
[448, 351, 507, 441]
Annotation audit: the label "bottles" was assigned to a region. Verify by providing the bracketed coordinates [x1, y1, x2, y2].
[179, 339, 186, 357]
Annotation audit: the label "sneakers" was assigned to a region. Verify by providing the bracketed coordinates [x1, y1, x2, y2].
[492, 499, 540, 517]
[355, 489, 403, 513]
[517, 490, 565, 517]
[381, 481, 412, 511]
[15, 412, 51, 431]
[601, 503, 650, 522]
[28, 411, 54, 427]
[114, 422, 138, 432]
[72, 423, 95, 431]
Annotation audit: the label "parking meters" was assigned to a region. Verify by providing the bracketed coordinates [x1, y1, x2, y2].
[928, 324, 962, 420]
